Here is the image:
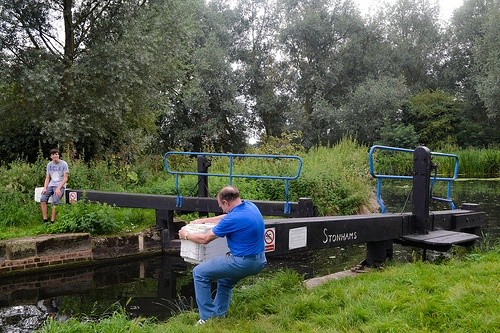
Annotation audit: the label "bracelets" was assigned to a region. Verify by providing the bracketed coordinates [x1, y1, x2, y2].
[184, 232, 190, 241]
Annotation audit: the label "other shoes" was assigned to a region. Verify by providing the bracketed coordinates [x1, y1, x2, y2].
[194, 318, 209, 325]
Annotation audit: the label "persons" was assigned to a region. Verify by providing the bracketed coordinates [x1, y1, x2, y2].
[178, 185, 268, 328]
[40, 149, 70, 224]
